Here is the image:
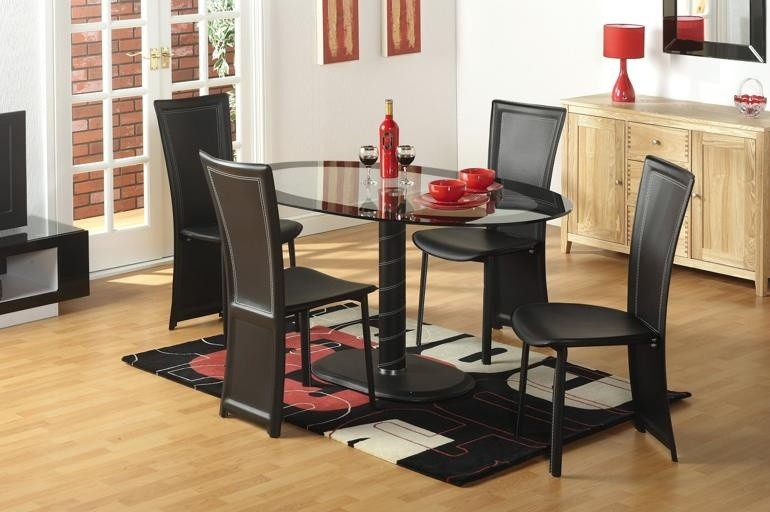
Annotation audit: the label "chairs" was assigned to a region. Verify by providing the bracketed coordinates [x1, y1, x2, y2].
[153, 92, 305, 333]
[513, 153, 696, 475]
[196, 148, 379, 441]
[409, 97, 567, 367]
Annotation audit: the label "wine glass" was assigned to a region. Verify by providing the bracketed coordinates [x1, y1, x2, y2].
[395, 145, 416, 185]
[359, 145, 379, 185]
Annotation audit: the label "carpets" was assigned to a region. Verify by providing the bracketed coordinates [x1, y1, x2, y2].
[121, 299, 694, 489]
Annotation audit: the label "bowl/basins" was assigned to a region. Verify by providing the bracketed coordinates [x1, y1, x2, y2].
[459, 168, 495, 190]
[428, 178, 466, 202]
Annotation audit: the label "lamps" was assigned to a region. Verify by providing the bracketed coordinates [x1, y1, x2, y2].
[602, 22, 645, 103]
[675, 15, 704, 43]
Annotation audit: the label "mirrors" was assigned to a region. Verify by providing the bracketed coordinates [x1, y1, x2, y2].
[662, 0, 768, 65]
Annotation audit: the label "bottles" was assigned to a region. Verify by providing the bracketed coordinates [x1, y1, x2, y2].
[380, 98, 400, 178]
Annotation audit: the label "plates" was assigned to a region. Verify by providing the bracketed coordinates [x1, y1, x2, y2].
[463, 182, 503, 194]
[414, 193, 490, 210]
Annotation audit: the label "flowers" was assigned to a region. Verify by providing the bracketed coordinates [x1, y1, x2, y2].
[694, 1, 706, 15]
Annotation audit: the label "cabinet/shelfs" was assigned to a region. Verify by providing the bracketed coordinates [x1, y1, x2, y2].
[559, 92, 770, 300]
[0, 213, 92, 330]
